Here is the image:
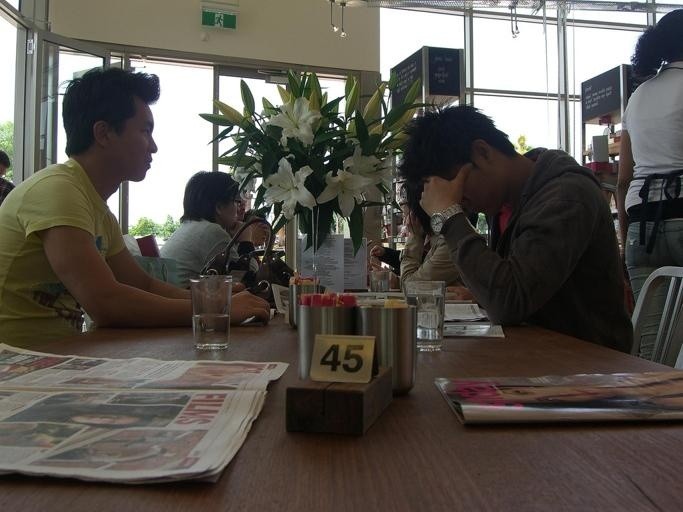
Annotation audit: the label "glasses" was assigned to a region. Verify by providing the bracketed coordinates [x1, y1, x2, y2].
[231, 199, 242, 207]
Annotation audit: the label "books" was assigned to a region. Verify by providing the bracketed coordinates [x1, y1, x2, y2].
[436, 371, 683, 425]
[443, 292, 507, 339]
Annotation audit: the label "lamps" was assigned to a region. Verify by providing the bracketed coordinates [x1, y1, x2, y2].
[330, 0, 348, 38]
[511, 0, 520, 38]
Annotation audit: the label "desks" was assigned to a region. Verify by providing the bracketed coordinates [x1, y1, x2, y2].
[0, 321, 683, 512]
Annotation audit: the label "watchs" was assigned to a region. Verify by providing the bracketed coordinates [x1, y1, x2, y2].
[429, 204, 463, 233]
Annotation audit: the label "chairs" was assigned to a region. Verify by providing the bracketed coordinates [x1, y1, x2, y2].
[629, 265, 683, 369]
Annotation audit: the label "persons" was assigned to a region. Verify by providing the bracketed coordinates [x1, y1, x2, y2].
[224, 201, 247, 233]
[399, 180, 465, 292]
[0, 66, 272, 326]
[157, 172, 258, 287]
[0, 150, 15, 205]
[244, 209, 268, 265]
[614, 9, 683, 368]
[398, 105, 641, 358]
[369, 244, 401, 275]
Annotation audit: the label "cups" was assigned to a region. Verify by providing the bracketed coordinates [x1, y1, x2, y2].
[403, 280, 446, 352]
[369, 270, 389, 291]
[189, 275, 232, 351]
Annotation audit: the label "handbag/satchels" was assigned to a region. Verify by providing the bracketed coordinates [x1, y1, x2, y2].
[200, 217, 295, 308]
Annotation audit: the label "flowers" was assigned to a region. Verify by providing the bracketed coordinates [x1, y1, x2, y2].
[199, 64, 437, 257]
